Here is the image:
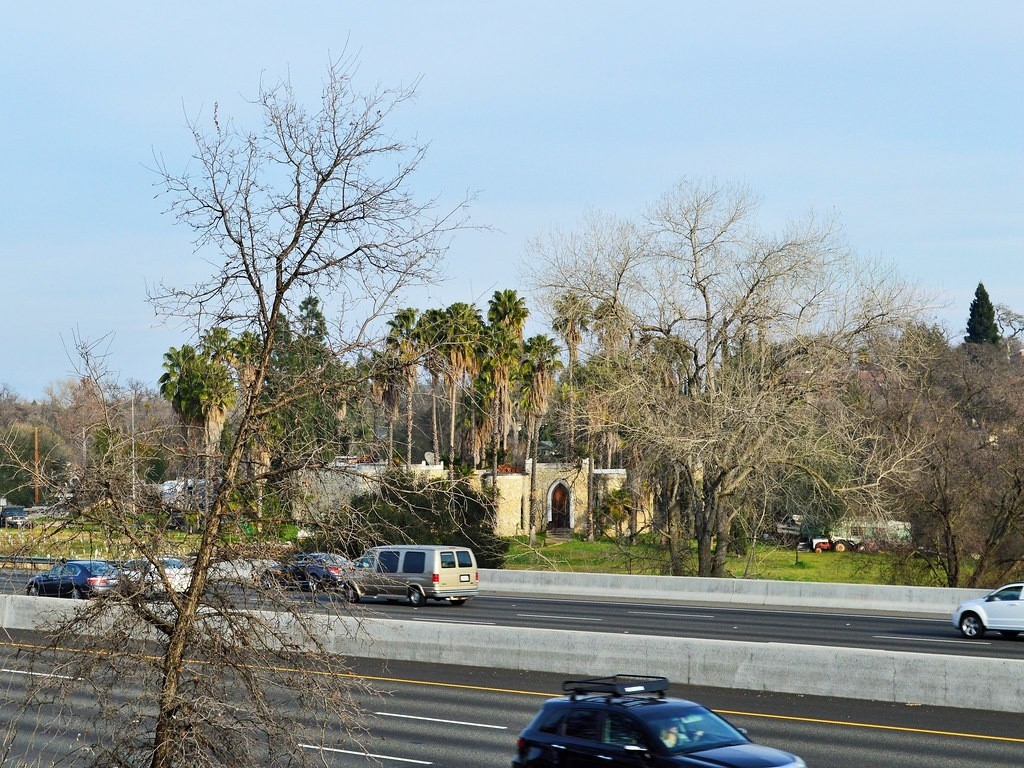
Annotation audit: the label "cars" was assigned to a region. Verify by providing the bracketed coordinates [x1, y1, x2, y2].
[259, 553, 354, 592]
[119, 556, 192, 600]
[951, 582, 1024, 640]
[24, 559, 131, 605]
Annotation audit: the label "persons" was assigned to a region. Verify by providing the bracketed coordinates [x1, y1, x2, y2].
[660, 725, 704, 748]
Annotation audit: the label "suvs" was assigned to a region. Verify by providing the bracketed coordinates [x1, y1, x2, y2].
[340, 543, 480, 608]
[0, 506, 31, 529]
[510, 674, 811, 768]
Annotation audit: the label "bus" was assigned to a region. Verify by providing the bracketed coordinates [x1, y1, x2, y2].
[160, 477, 223, 514]
